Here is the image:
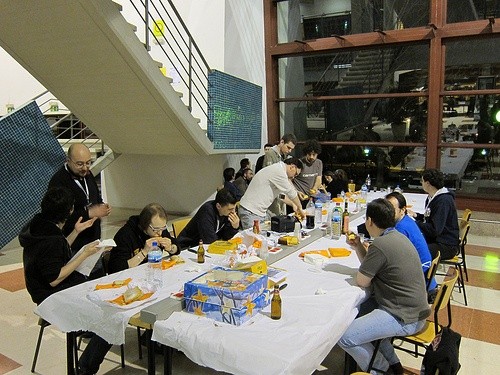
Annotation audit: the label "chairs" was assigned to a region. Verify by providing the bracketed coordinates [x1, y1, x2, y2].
[425, 251, 441, 293]
[464, 139, 494, 179]
[362, 266, 459, 373]
[441, 219, 471, 306]
[171, 217, 191, 238]
[461, 208, 472, 247]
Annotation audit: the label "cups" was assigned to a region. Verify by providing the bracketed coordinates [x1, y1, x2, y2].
[357, 233, 365, 243]
[144, 268, 154, 282]
[225, 250, 233, 262]
[313, 258, 323, 273]
[346, 226, 356, 240]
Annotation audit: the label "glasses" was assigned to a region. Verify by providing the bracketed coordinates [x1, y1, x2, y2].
[148, 224, 167, 231]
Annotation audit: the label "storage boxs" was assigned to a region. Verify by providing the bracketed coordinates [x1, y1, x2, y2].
[186, 267, 268, 311]
[184, 290, 271, 327]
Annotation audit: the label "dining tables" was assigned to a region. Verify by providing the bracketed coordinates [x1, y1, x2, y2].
[33, 186, 428, 375]
[387, 135, 474, 190]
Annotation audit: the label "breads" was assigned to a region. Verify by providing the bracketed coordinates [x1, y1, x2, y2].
[329, 248, 351, 257]
[348, 234, 356, 239]
[122, 287, 142, 304]
[112, 280, 124, 286]
[299, 250, 329, 257]
[278, 236, 298, 245]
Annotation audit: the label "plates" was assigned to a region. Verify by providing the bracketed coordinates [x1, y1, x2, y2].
[88, 285, 159, 309]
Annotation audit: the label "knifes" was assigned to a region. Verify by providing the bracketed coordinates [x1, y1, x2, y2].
[270, 284, 287, 294]
[188, 249, 212, 258]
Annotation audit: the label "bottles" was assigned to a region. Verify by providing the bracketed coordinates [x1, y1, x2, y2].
[305, 179, 367, 240]
[197, 239, 205, 263]
[253, 220, 259, 234]
[394, 185, 402, 194]
[271, 285, 282, 320]
[147, 242, 163, 289]
[365, 174, 371, 192]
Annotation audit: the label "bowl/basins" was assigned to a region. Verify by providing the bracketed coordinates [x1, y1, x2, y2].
[261, 231, 280, 246]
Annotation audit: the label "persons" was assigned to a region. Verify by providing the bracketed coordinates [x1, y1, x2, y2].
[468, 122, 500, 162]
[337, 198, 429, 375]
[407, 168, 460, 261]
[177, 188, 241, 249]
[49, 143, 112, 280]
[19, 186, 114, 375]
[107, 202, 180, 348]
[223, 134, 323, 230]
[385, 192, 437, 303]
[318, 170, 345, 200]
[319, 124, 423, 167]
